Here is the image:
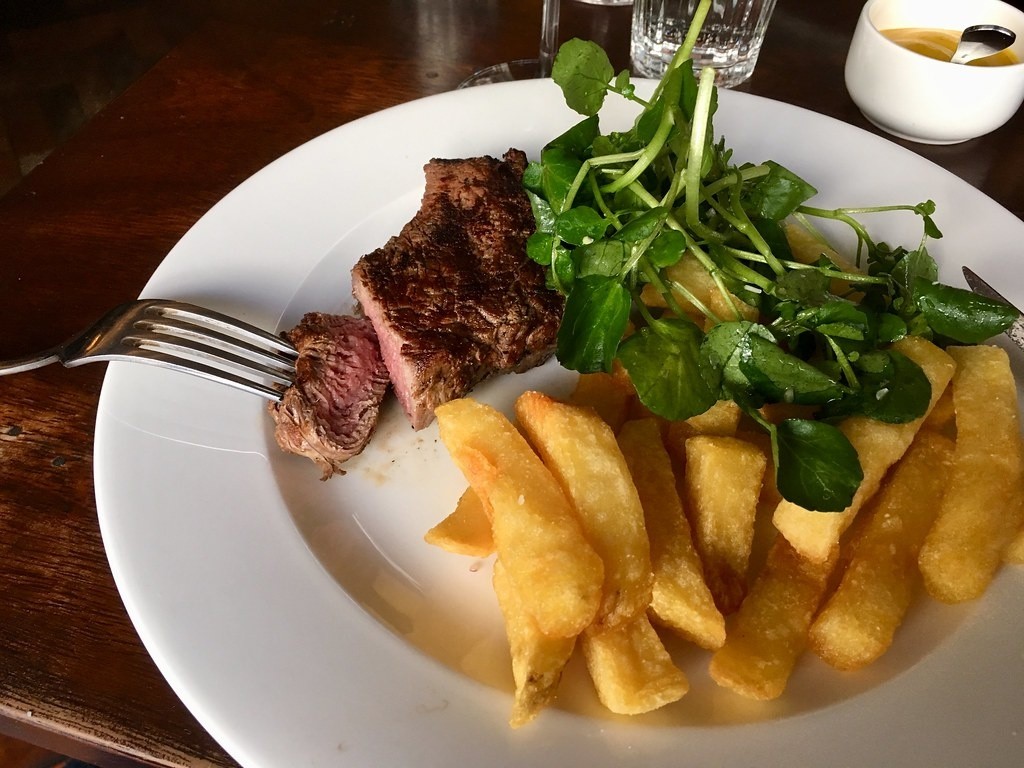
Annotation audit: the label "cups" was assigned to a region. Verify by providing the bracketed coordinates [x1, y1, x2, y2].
[629, 1, 775, 89]
[846, 1, 1024, 148]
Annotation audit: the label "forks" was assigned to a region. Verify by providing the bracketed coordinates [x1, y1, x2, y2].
[2, 298, 302, 407]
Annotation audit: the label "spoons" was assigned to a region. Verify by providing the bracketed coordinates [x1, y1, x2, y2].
[950, 25, 1015, 65]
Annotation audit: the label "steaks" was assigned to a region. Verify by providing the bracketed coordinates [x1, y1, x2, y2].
[267, 148, 571, 480]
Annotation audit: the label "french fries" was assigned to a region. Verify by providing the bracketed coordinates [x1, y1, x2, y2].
[431, 240, 1024, 730]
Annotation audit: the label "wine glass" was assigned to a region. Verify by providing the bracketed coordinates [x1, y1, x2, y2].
[456, 0, 564, 91]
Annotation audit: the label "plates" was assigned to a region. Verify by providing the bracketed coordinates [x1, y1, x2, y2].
[96, 77, 1024, 768]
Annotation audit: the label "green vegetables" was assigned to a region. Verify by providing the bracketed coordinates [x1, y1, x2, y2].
[522, 0, 1010, 514]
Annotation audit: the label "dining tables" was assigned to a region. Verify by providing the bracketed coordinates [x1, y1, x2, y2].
[0, 0, 1024, 768]
